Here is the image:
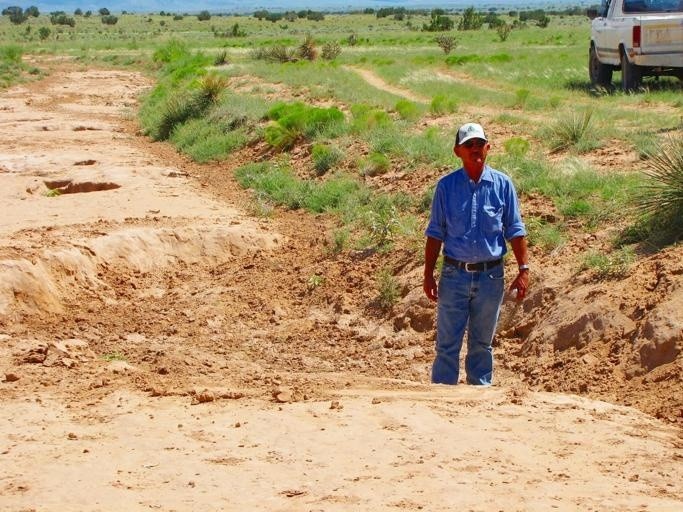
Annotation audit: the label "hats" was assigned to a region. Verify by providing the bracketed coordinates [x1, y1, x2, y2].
[456, 123, 487, 146]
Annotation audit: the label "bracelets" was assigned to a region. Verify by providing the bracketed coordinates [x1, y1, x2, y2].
[519, 265, 529, 270]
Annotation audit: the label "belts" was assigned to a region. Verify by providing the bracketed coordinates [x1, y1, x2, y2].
[444, 256, 502, 273]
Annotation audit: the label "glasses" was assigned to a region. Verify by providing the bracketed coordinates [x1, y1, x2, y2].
[464, 139, 484, 148]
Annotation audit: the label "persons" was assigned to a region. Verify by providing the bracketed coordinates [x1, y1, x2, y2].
[423, 122, 530, 385]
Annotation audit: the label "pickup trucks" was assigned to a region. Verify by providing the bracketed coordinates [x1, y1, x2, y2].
[588, 0, 683, 92]
